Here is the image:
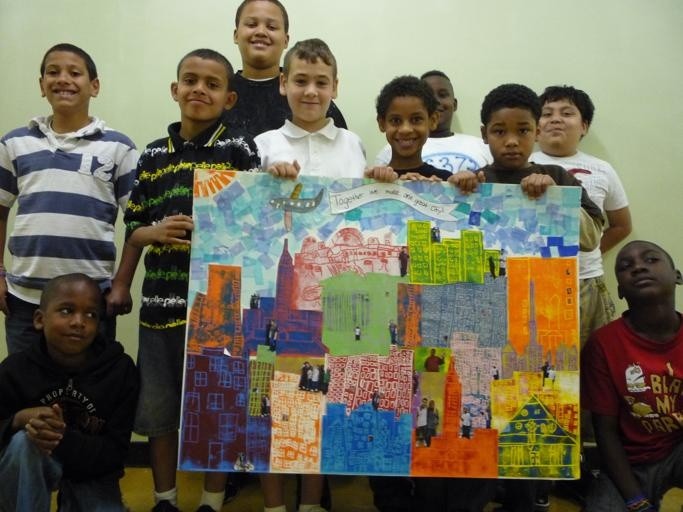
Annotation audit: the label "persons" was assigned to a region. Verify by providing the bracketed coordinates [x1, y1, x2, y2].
[263, 318, 280, 351]
[527, 85, 633, 512]
[580, 239, 683, 512]
[459, 405, 491, 440]
[261, 394, 271, 418]
[0, 42, 144, 355]
[298, 361, 331, 395]
[249, 226, 508, 311]
[447, 82, 606, 512]
[122, 48, 264, 512]
[492, 365, 500, 380]
[223, 0, 348, 512]
[373, 69, 493, 512]
[423, 348, 445, 372]
[540, 360, 556, 387]
[254, 37, 399, 512]
[0, 271, 138, 512]
[353, 326, 362, 341]
[368, 74, 455, 512]
[387, 319, 398, 345]
